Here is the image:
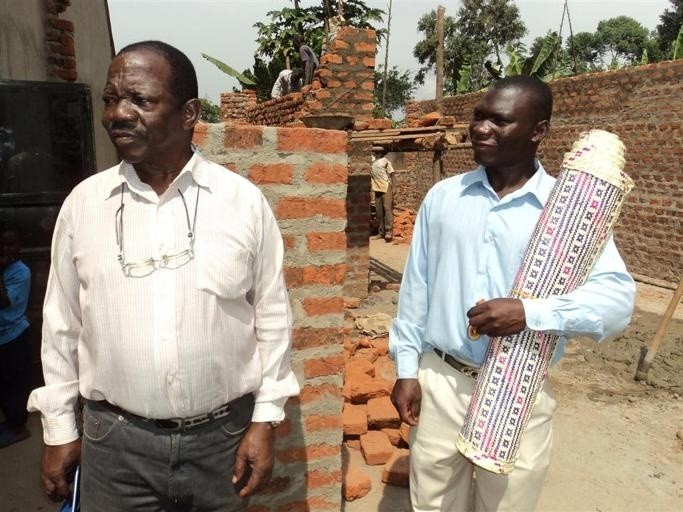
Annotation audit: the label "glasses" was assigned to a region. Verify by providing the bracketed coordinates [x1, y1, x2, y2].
[114, 188, 194, 267]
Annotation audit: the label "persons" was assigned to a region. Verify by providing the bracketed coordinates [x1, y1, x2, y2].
[26, 40, 302, 512]
[371, 150, 398, 240]
[270, 33, 319, 102]
[388, 76, 636, 512]
[0, 229, 33, 446]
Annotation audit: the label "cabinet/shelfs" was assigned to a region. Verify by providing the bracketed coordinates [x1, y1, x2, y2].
[0, 79, 98, 384]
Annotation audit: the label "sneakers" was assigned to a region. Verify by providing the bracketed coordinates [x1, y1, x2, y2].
[371, 232, 392, 241]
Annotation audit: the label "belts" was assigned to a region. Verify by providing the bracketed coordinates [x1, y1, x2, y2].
[98, 400, 231, 430]
[433, 348, 478, 379]
